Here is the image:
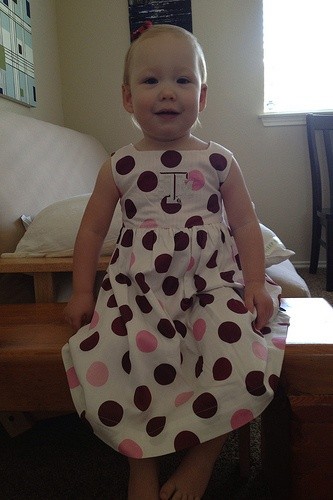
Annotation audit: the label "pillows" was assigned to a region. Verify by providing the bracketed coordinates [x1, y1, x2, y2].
[14, 193, 297, 269]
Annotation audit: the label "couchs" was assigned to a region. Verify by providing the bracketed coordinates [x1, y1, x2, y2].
[1, 111, 309, 302]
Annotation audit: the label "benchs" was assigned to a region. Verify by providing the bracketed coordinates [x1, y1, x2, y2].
[1, 298, 332, 500]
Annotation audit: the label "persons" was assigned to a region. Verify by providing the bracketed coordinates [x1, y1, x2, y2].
[62, 25, 290, 500]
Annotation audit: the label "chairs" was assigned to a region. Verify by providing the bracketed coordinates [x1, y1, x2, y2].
[305, 113, 333, 293]
